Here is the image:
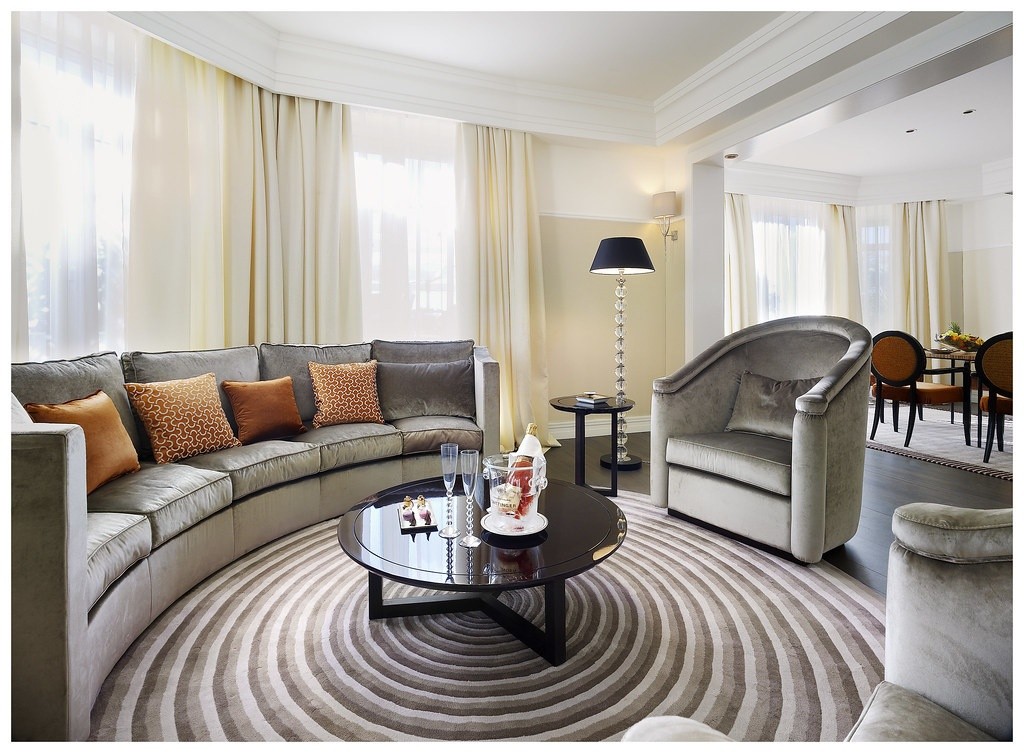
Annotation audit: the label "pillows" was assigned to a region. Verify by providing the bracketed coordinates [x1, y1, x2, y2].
[123, 371, 242, 464]
[220, 376, 308, 444]
[376, 355, 477, 423]
[23, 389, 141, 495]
[307, 359, 386, 429]
[726, 367, 824, 442]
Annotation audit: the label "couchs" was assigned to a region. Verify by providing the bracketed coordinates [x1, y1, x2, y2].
[650, 315, 874, 566]
[622, 502, 1013, 742]
[11, 340, 501, 743]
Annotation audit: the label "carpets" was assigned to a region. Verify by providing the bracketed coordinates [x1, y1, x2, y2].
[86, 482, 889, 741]
[865, 402, 1013, 482]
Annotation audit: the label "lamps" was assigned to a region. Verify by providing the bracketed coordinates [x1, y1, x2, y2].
[652, 191, 679, 240]
[589, 236, 655, 473]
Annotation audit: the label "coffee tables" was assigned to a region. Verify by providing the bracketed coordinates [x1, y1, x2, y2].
[337, 474, 627, 667]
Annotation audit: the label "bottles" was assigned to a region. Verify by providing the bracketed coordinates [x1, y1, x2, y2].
[505, 423, 540, 517]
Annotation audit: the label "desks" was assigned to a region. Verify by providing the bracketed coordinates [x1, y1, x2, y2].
[550, 395, 635, 497]
[924, 349, 978, 446]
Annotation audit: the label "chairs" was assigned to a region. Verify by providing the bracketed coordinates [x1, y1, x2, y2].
[980, 330, 1013, 462]
[870, 330, 963, 447]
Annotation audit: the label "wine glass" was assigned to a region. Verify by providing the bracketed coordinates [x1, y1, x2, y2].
[438, 443, 462, 538]
[460, 450, 481, 548]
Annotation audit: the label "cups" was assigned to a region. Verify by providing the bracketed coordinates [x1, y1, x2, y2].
[484, 453, 543, 530]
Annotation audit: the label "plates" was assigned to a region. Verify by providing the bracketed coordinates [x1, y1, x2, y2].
[480, 511, 548, 536]
